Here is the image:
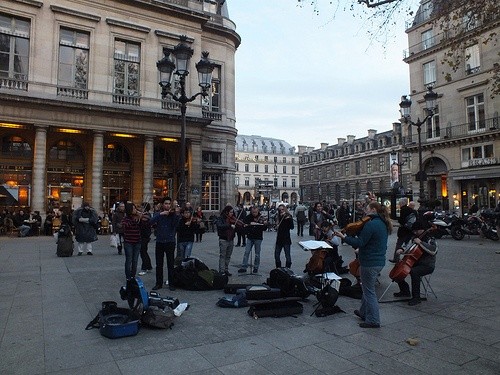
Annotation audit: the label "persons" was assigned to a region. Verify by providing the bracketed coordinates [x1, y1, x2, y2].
[238, 206, 268, 272]
[107, 200, 153, 280]
[334, 201, 392, 328]
[274, 204, 295, 268]
[309, 200, 351, 245]
[388, 198, 421, 263]
[43, 212, 56, 236]
[235, 206, 247, 247]
[320, 221, 339, 248]
[60, 202, 102, 256]
[149, 197, 180, 291]
[295, 201, 307, 237]
[216, 205, 236, 276]
[393, 221, 438, 306]
[350, 194, 373, 223]
[172, 199, 205, 258]
[0, 209, 42, 236]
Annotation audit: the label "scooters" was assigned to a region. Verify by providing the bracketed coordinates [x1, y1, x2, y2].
[422, 205, 499, 241]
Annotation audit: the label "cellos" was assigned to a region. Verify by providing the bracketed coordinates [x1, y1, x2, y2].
[305, 231, 337, 274]
[378, 226, 439, 302]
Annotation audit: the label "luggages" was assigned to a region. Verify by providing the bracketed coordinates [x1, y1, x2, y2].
[56, 237, 74, 257]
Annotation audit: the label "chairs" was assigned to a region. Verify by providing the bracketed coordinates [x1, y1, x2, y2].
[6, 218, 22, 238]
[100, 218, 109, 234]
[51, 218, 62, 233]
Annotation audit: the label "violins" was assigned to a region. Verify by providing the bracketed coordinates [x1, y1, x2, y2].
[188, 217, 215, 225]
[255, 214, 270, 223]
[166, 205, 184, 215]
[132, 211, 151, 221]
[227, 214, 246, 225]
[329, 217, 371, 241]
[278, 213, 297, 222]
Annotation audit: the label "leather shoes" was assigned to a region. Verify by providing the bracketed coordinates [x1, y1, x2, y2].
[152, 284, 162, 290]
[169, 285, 176, 290]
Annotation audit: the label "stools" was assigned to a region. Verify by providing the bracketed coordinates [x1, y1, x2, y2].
[419, 267, 437, 300]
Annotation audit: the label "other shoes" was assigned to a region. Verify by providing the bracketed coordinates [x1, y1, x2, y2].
[145, 269, 153, 273]
[87, 252, 93, 255]
[225, 271, 232, 276]
[78, 252, 82, 256]
[238, 269, 247, 273]
[393, 289, 410, 297]
[359, 320, 380, 328]
[138, 271, 146, 276]
[389, 259, 399, 263]
[253, 270, 257, 273]
[354, 309, 365, 321]
[408, 297, 421, 306]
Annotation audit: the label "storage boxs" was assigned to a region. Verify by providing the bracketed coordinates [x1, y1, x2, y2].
[423, 211, 436, 221]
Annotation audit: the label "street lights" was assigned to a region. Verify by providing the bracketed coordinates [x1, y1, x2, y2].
[399, 85, 439, 200]
[264, 182, 272, 221]
[155, 34, 216, 210]
